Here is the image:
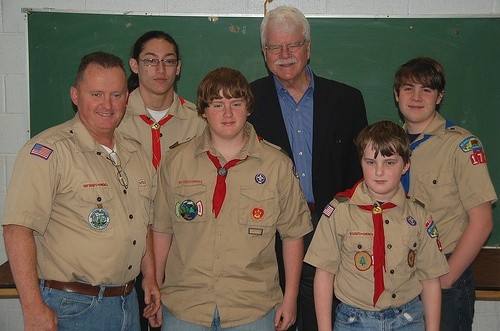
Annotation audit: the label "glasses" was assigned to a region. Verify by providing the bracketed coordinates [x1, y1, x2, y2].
[137, 57, 181, 68]
[107, 152, 129, 190]
[264, 40, 306, 54]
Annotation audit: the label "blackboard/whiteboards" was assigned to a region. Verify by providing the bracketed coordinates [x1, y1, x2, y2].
[16, 7, 500, 249]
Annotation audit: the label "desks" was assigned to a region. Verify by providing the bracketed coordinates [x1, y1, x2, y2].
[1, 248, 500, 331]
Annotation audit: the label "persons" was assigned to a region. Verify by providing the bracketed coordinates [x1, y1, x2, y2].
[383, 55, 499, 330]
[146, 67, 315, 331]
[244, 5, 369, 331]
[303, 121, 451, 331]
[114, 30, 210, 174]
[0, 51, 162, 330]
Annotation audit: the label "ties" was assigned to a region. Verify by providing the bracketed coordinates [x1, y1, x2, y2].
[359, 203, 397, 304]
[138, 114, 174, 171]
[400, 131, 432, 199]
[205, 151, 240, 218]
